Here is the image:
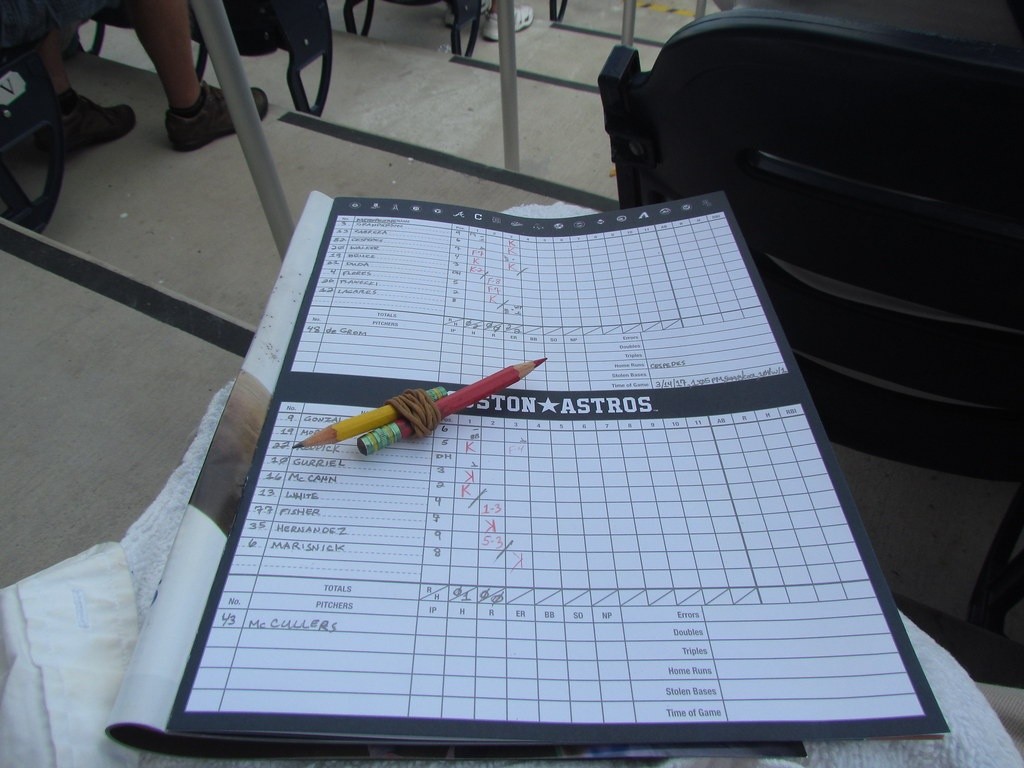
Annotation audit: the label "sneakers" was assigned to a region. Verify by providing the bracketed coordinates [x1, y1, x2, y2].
[443, 0, 492, 27]
[30, 95, 136, 157]
[482, 5, 535, 41]
[165, 80, 269, 152]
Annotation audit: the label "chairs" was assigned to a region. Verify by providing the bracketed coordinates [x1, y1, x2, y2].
[597, 7, 1024, 691]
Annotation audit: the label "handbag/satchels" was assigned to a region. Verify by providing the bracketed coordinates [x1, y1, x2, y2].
[0, 44, 66, 241]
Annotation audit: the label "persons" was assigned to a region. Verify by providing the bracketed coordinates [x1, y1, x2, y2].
[444, 0, 534, 42]
[0, 0, 268, 160]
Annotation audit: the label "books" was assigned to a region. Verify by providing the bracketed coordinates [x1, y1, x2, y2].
[104, 191, 951, 758]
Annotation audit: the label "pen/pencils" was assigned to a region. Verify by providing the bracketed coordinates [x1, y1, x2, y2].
[356, 357, 548, 456]
[292, 387, 447, 449]
